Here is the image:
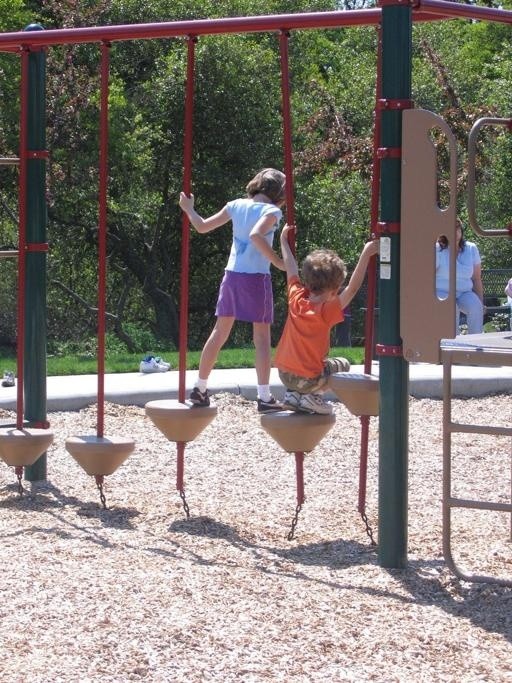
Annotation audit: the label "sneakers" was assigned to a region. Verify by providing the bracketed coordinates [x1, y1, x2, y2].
[155, 357, 172, 370]
[188, 386, 211, 406]
[139, 356, 169, 372]
[256, 394, 288, 414]
[297, 394, 334, 415]
[278, 388, 302, 411]
[1, 370, 16, 388]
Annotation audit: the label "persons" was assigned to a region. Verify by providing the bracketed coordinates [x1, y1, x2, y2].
[178, 168, 299, 414]
[273, 221, 378, 414]
[436, 218, 483, 334]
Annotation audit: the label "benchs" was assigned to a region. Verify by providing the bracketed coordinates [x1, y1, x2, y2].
[346, 269, 512, 360]
